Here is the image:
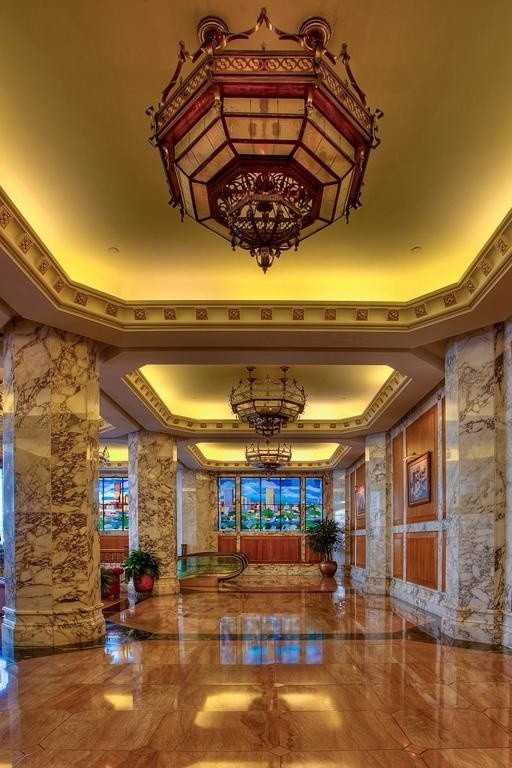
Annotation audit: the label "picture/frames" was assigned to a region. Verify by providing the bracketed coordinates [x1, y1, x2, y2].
[354, 485, 365, 519]
[404, 450, 431, 507]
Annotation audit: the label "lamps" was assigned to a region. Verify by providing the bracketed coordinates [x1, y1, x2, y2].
[229, 363, 308, 484]
[142, 6, 384, 277]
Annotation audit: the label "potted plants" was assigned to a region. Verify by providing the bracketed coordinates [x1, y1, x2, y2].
[304, 520, 342, 577]
[123, 549, 162, 593]
